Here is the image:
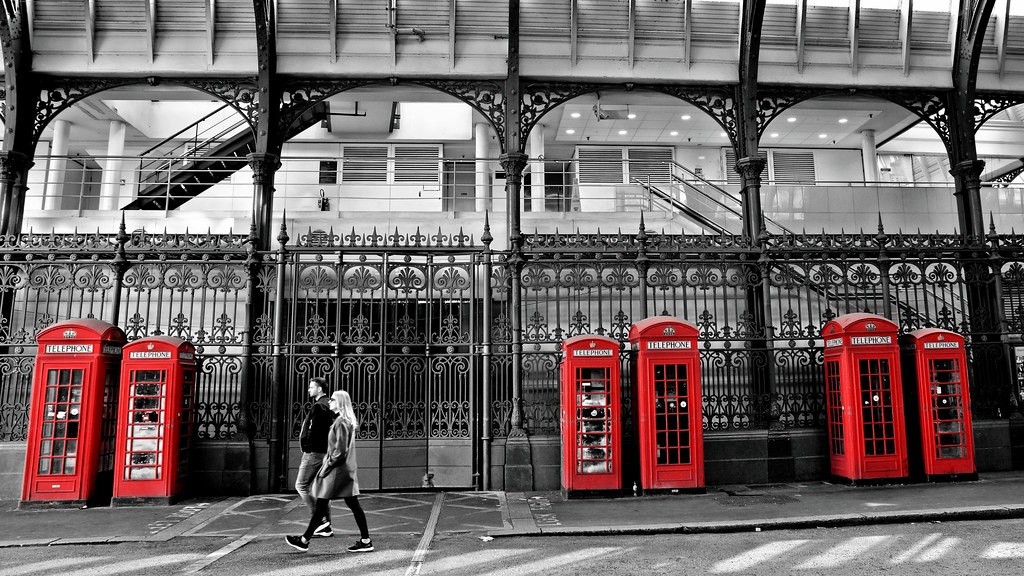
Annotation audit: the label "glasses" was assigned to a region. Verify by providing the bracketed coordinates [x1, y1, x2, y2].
[330, 397, 335, 402]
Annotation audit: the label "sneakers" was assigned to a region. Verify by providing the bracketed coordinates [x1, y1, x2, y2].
[314, 516, 330, 532]
[313, 526, 333, 537]
[347, 539, 374, 552]
[285, 535, 310, 552]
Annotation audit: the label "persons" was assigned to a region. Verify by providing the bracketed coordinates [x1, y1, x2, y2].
[285, 390, 374, 551]
[295, 378, 340, 537]
